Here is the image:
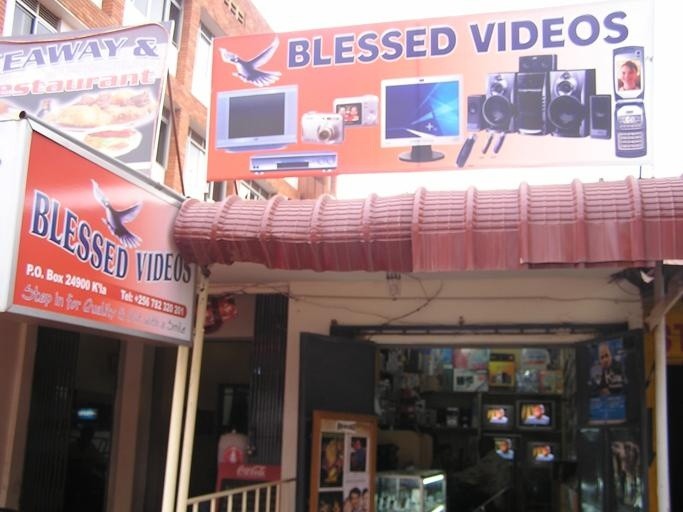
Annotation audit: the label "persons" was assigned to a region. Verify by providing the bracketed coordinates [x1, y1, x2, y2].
[617, 59, 639, 89]
[491, 407, 507, 422]
[526, 403, 551, 423]
[320, 437, 368, 511]
[337, 105, 349, 121]
[533, 445, 553, 461]
[587, 342, 625, 397]
[495, 438, 515, 461]
[347, 107, 358, 122]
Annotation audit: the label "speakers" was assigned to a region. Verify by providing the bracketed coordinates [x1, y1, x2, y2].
[589, 95, 611, 140]
[547, 69, 596, 137]
[467, 95, 486, 131]
[519, 55, 557, 73]
[482, 72, 517, 133]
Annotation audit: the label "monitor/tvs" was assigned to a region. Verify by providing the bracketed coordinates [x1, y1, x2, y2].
[527, 441, 561, 468]
[516, 399, 556, 431]
[214, 84, 298, 152]
[380, 74, 464, 162]
[482, 432, 522, 469]
[482, 405, 515, 432]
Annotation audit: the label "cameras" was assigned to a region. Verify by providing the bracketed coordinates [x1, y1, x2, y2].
[334, 95, 378, 127]
[301, 113, 344, 144]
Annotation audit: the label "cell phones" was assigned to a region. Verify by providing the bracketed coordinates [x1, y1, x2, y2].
[612, 46, 647, 157]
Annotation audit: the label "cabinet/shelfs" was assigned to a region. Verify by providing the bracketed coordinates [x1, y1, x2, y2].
[375, 468, 448, 512]
